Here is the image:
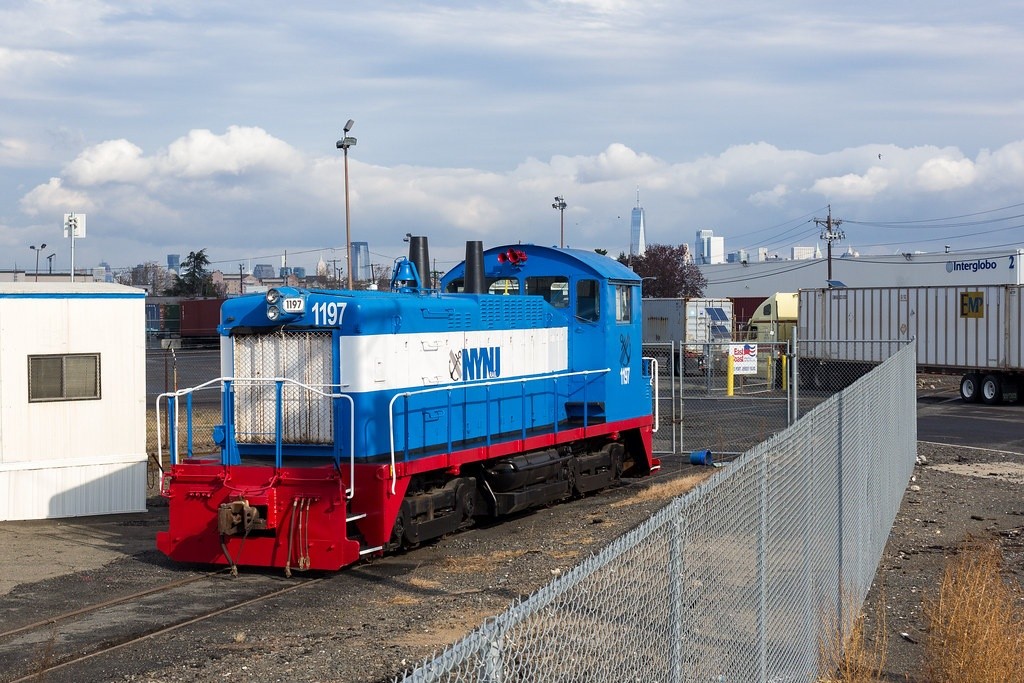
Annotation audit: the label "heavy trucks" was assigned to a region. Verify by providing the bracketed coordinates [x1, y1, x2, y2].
[745, 283, 1024, 405]
[642, 297, 734, 376]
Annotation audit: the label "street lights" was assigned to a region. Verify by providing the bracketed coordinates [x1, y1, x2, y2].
[553, 195, 567, 248]
[336, 119, 357, 290]
[30, 244, 48, 282]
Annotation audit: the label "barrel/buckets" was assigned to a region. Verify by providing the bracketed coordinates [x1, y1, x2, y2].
[690, 450, 713, 465]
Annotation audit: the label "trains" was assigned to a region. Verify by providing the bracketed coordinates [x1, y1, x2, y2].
[150, 237, 660, 577]
